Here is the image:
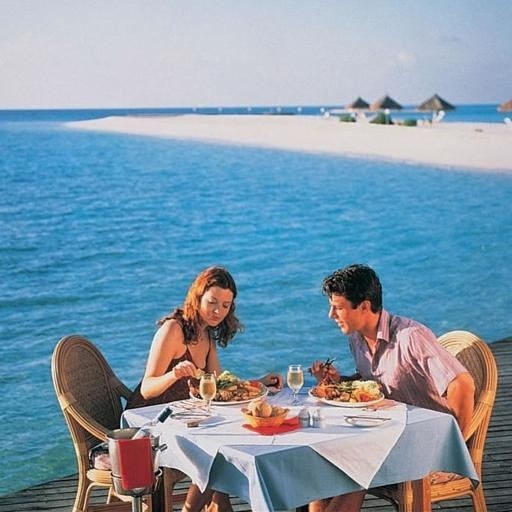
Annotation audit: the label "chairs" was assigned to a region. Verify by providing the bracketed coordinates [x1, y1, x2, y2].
[51, 335, 186, 512]
[366, 330, 498, 512]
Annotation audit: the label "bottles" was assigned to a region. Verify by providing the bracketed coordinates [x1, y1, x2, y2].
[132, 407, 176, 440]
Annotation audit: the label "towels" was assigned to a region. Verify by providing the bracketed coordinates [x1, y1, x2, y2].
[169, 400, 206, 410]
[267, 384, 281, 395]
[117, 438, 156, 491]
[366, 398, 397, 411]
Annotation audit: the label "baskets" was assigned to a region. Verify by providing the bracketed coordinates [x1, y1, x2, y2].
[240, 407, 289, 427]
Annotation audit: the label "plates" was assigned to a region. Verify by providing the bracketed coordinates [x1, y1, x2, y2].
[308, 387, 385, 408]
[188, 382, 269, 405]
[344, 414, 387, 427]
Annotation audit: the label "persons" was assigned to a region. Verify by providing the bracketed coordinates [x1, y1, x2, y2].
[309, 265, 476, 512]
[120, 266, 283, 512]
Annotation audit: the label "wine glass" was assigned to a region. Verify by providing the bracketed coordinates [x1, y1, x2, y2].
[199, 374, 216, 416]
[287, 365, 304, 406]
